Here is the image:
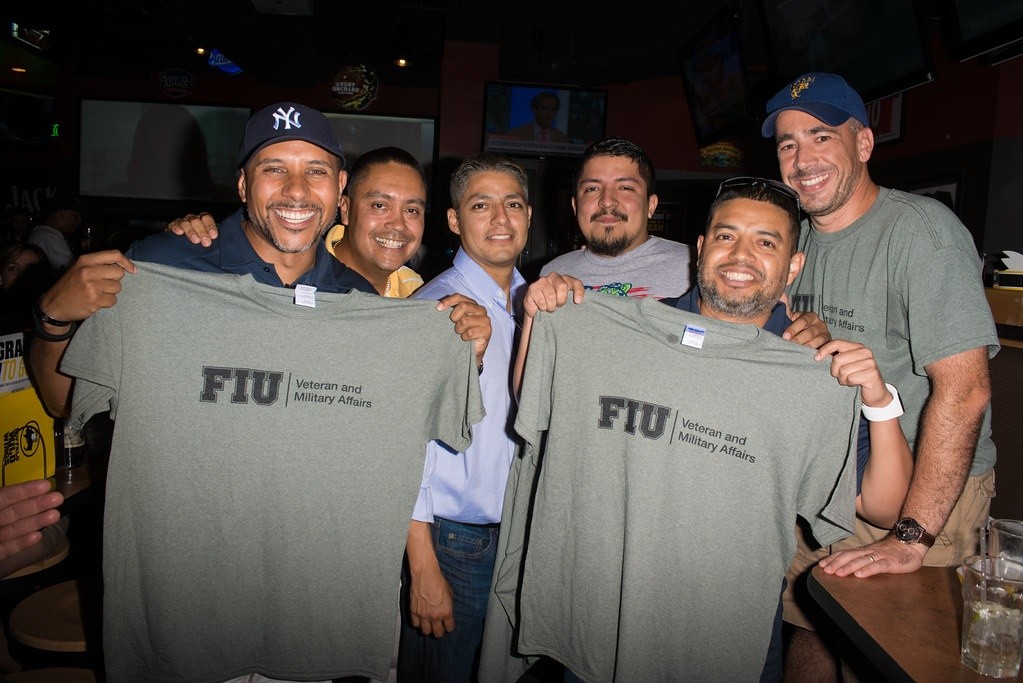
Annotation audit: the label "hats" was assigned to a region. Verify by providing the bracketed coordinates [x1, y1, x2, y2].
[762, 73, 869, 137]
[237, 102, 346, 178]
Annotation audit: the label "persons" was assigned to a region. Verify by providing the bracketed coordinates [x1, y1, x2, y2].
[0, 479, 65, 559]
[163, 147, 424, 301]
[512, 177, 914, 683]
[30, 101, 493, 683]
[506, 92, 572, 143]
[761, 71, 1003, 683]
[396, 154, 533, 682]
[4, 190, 89, 282]
[0, 241, 54, 338]
[538, 137, 699, 299]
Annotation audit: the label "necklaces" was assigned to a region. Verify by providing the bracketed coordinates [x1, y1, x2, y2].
[333, 237, 391, 298]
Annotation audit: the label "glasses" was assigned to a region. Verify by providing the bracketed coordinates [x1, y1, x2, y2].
[716, 177, 800, 220]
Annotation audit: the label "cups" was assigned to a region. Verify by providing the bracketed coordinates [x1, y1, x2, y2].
[960, 555, 1022, 679]
[987, 520, 1022, 560]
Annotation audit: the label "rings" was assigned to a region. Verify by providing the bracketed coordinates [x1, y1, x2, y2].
[865, 555, 875, 562]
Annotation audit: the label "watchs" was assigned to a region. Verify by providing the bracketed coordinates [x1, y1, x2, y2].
[892, 516, 937, 548]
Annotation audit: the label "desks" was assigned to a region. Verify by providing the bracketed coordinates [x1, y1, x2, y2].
[791, 563, 1021, 682]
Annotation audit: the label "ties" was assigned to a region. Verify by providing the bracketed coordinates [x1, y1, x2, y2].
[539, 130, 548, 142]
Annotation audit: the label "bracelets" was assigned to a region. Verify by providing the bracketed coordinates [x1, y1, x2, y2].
[35, 292, 74, 327]
[30, 300, 77, 343]
[860, 381, 905, 422]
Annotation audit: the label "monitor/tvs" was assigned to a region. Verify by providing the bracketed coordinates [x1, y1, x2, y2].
[481, 80, 609, 161]
